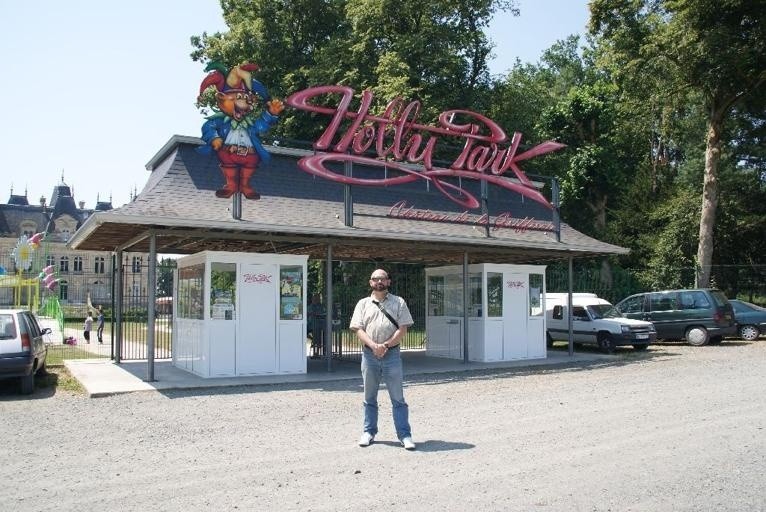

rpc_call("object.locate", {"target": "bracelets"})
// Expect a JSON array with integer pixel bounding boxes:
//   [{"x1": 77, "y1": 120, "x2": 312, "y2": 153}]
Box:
[{"x1": 383, "y1": 343, "x2": 389, "y2": 349}]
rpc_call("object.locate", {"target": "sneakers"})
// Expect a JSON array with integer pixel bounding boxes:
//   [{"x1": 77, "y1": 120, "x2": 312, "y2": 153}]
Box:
[
  {"x1": 359, "y1": 432, "x2": 375, "y2": 446},
  {"x1": 400, "y1": 436, "x2": 416, "y2": 450}
]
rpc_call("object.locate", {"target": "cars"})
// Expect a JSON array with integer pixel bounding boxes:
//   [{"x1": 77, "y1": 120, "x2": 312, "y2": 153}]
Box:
[
  {"x1": 728, "y1": 300, "x2": 766, "y2": 340},
  {"x1": 0, "y1": 310, "x2": 52, "y2": 395}
]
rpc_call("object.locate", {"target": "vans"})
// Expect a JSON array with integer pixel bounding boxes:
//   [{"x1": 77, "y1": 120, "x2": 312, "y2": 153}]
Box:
[{"x1": 546, "y1": 288, "x2": 739, "y2": 353}]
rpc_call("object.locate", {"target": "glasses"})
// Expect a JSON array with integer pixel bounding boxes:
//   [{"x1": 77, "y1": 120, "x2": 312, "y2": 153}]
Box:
[{"x1": 368, "y1": 276, "x2": 389, "y2": 283}]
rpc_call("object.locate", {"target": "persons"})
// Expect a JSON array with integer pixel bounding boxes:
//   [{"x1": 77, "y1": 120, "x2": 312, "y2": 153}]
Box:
[
  {"x1": 307, "y1": 292, "x2": 326, "y2": 347},
  {"x1": 348, "y1": 268, "x2": 416, "y2": 450},
  {"x1": 82, "y1": 311, "x2": 94, "y2": 344},
  {"x1": 96, "y1": 310, "x2": 104, "y2": 345}
]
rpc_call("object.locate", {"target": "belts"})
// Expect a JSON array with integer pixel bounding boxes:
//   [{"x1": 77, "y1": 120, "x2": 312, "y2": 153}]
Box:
[{"x1": 363, "y1": 343, "x2": 400, "y2": 350}]
